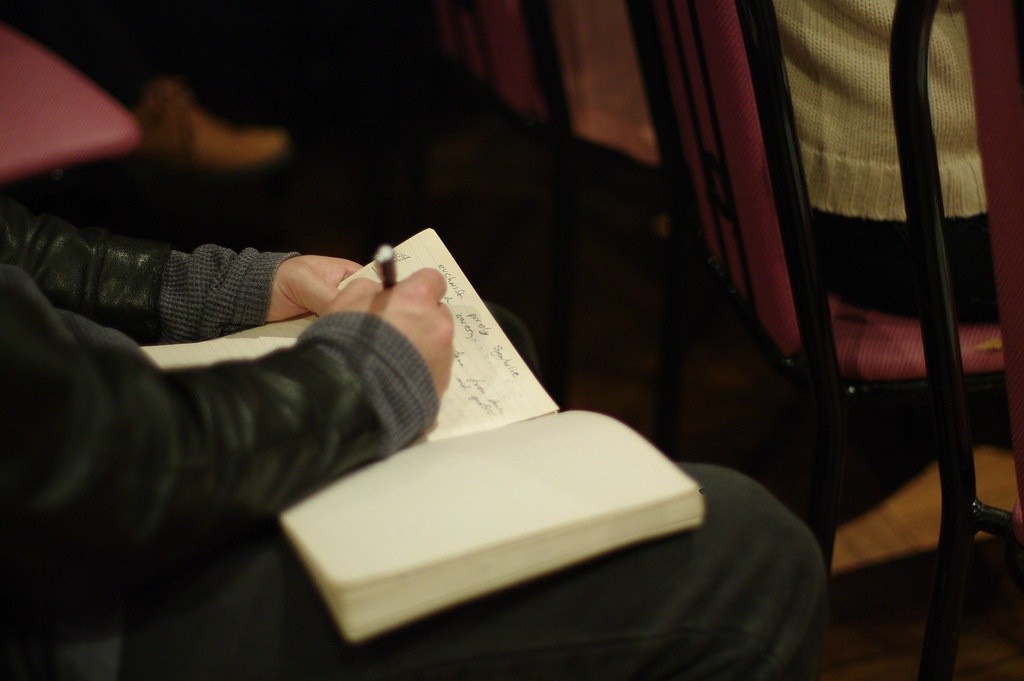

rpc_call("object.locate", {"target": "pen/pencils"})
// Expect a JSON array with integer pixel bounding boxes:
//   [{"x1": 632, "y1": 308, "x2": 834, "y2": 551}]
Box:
[{"x1": 373, "y1": 245, "x2": 398, "y2": 291}]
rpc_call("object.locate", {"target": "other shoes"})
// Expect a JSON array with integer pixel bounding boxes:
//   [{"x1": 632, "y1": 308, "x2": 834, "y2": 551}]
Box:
[{"x1": 120, "y1": 77, "x2": 289, "y2": 174}]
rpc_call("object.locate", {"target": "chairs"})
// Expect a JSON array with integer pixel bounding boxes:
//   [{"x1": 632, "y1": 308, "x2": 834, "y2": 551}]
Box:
[{"x1": 363, "y1": 0, "x2": 1024, "y2": 681}]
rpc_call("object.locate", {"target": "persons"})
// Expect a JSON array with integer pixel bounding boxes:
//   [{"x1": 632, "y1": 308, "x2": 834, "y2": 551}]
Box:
[
  {"x1": 774, "y1": 0, "x2": 1000, "y2": 324},
  {"x1": 0, "y1": 197, "x2": 828, "y2": 681}
]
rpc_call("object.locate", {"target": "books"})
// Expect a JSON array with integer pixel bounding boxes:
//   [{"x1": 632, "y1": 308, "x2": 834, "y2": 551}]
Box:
[{"x1": 141, "y1": 227, "x2": 704, "y2": 642}]
